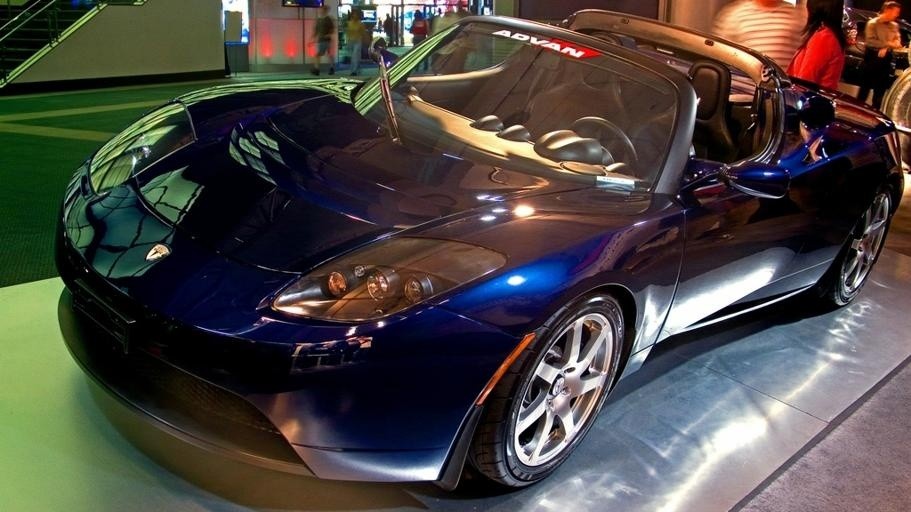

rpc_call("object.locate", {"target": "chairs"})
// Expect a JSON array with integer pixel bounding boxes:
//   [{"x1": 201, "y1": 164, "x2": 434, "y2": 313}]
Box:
[{"x1": 521, "y1": 32, "x2": 737, "y2": 179}]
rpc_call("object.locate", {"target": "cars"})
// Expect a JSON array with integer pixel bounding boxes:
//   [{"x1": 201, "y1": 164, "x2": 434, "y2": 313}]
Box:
[{"x1": 841, "y1": 4, "x2": 911, "y2": 77}]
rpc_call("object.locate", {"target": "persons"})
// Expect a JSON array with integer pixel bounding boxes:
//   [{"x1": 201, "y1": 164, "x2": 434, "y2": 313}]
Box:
[
  {"x1": 857, "y1": 1, "x2": 903, "y2": 112},
  {"x1": 711, "y1": 0, "x2": 809, "y2": 74},
  {"x1": 377, "y1": 0, "x2": 472, "y2": 75},
  {"x1": 308, "y1": 5, "x2": 336, "y2": 75},
  {"x1": 784, "y1": 0, "x2": 849, "y2": 91},
  {"x1": 344, "y1": 8, "x2": 365, "y2": 76}
]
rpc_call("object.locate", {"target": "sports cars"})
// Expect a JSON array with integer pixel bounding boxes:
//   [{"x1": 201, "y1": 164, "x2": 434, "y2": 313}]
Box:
[{"x1": 54, "y1": 9, "x2": 904, "y2": 491}]
